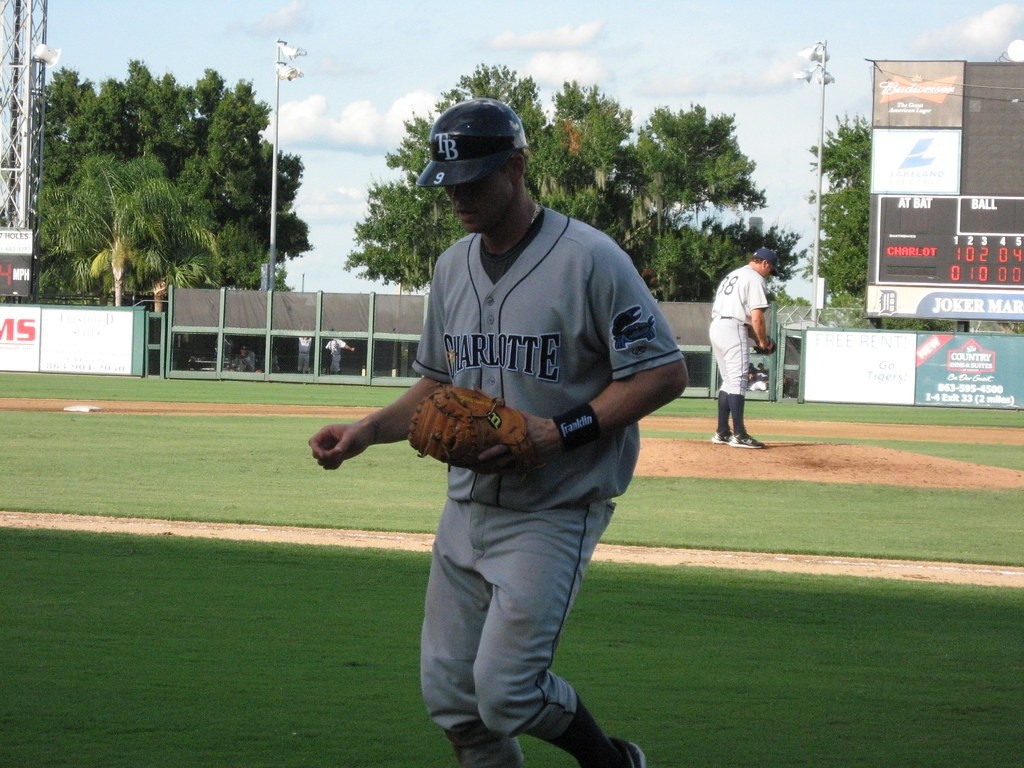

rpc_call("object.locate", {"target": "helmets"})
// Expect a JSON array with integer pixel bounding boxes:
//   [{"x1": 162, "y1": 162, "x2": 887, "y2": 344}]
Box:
[{"x1": 415, "y1": 98, "x2": 528, "y2": 187}]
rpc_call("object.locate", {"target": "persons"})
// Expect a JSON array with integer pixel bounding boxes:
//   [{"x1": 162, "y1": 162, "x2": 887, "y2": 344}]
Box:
[
  {"x1": 325, "y1": 338, "x2": 355, "y2": 374},
  {"x1": 297, "y1": 337, "x2": 312, "y2": 373},
  {"x1": 709, "y1": 247, "x2": 782, "y2": 449},
  {"x1": 239, "y1": 345, "x2": 248, "y2": 356},
  {"x1": 307, "y1": 99, "x2": 690, "y2": 768},
  {"x1": 746, "y1": 362, "x2": 769, "y2": 391}
]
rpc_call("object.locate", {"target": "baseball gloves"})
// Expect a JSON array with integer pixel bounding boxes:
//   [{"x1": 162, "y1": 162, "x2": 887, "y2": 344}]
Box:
[
  {"x1": 753, "y1": 336, "x2": 778, "y2": 356},
  {"x1": 406, "y1": 386, "x2": 537, "y2": 477}
]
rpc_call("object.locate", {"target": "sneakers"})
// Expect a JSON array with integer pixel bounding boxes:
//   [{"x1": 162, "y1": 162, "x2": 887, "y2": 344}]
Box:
[
  {"x1": 730, "y1": 434, "x2": 764, "y2": 448},
  {"x1": 712, "y1": 433, "x2": 733, "y2": 445}
]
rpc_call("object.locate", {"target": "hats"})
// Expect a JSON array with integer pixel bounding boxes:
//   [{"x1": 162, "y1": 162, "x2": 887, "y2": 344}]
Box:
[{"x1": 753, "y1": 249, "x2": 779, "y2": 276}]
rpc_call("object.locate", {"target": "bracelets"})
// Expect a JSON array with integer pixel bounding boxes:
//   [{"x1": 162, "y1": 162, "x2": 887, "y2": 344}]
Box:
[{"x1": 552, "y1": 403, "x2": 602, "y2": 453}]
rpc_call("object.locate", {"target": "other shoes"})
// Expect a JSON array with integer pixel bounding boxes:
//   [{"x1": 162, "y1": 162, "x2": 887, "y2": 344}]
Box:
[{"x1": 611, "y1": 737, "x2": 646, "y2": 768}]
